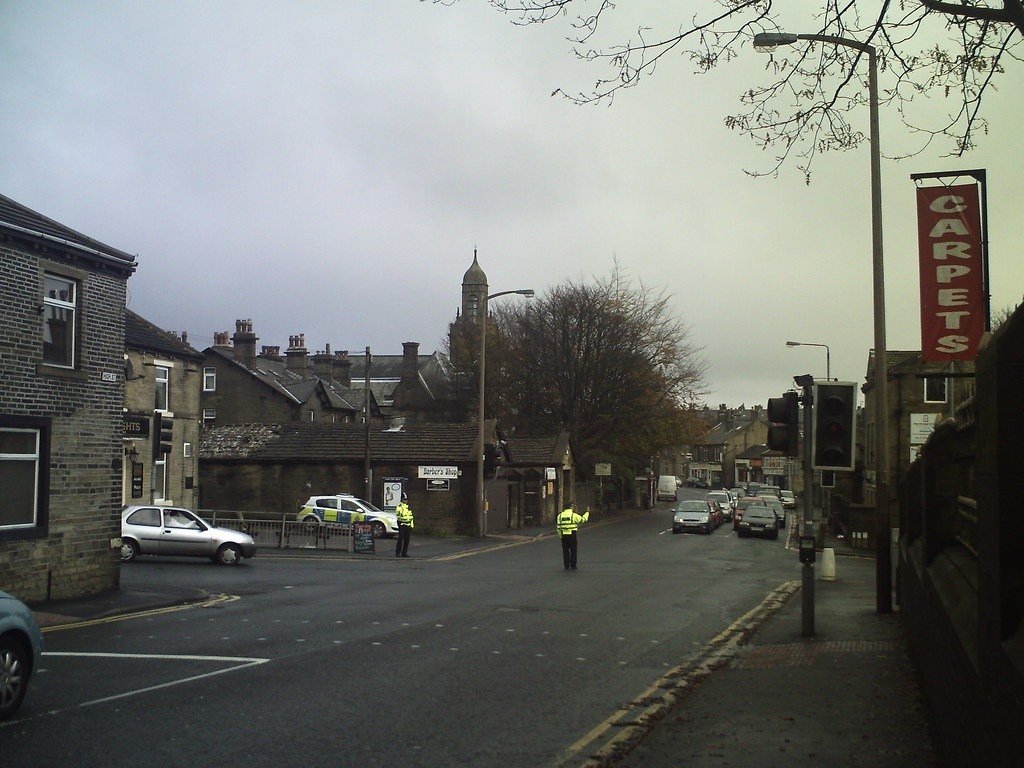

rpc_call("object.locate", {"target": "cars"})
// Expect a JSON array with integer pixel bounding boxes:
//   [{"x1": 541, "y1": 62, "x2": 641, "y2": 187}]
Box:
[
  {"x1": 297, "y1": 493, "x2": 400, "y2": 540},
  {"x1": 118, "y1": 505, "x2": 256, "y2": 567},
  {"x1": 0, "y1": 590, "x2": 45, "y2": 721},
  {"x1": 671, "y1": 481, "x2": 798, "y2": 539}
]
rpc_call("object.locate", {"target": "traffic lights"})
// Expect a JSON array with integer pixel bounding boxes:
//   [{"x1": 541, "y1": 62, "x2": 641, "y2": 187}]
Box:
[
  {"x1": 812, "y1": 382, "x2": 857, "y2": 470},
  {"x1": 766, "y1": 392, "x2": 799, "y2": 456}
]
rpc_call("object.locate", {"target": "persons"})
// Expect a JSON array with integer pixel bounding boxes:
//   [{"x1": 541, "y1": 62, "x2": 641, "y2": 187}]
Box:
[
  {"x1": 386, "y1": 487, "x2": 393, "y2": 505},
  {"x1": 557, "y1": 501, "x2": 590, "y2": 569},
  {"x1": 396, "y1": 493, "x2": 414, "y2": 557}
]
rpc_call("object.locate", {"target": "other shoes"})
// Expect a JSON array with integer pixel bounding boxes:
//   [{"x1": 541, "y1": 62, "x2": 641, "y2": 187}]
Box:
[
  {"x1": 565, "y1": 566, "x2": 577, "y2": 569},
  {"x1": 396, "y1": 555, "x2": 409, "y2": 558}
]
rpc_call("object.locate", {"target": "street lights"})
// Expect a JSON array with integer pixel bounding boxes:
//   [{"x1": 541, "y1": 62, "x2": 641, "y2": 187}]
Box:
[
  {"x1": 752, "y1": 32, "x2": 893, "y2": 615},
  {"x1": 473, "y1": 289, "x2": 536, "y2": 537},
  {"x1": 786, "y1": 341, "x2": 830, "y2": 381}
]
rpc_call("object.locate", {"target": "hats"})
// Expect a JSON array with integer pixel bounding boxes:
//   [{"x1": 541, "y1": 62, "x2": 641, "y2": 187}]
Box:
[
  {"x1": 564, "y1": 501, "x2": 573, "y2": 509},
  {"x1": 401, "y1": 493, "x2": 408, "y2": 500}
]
